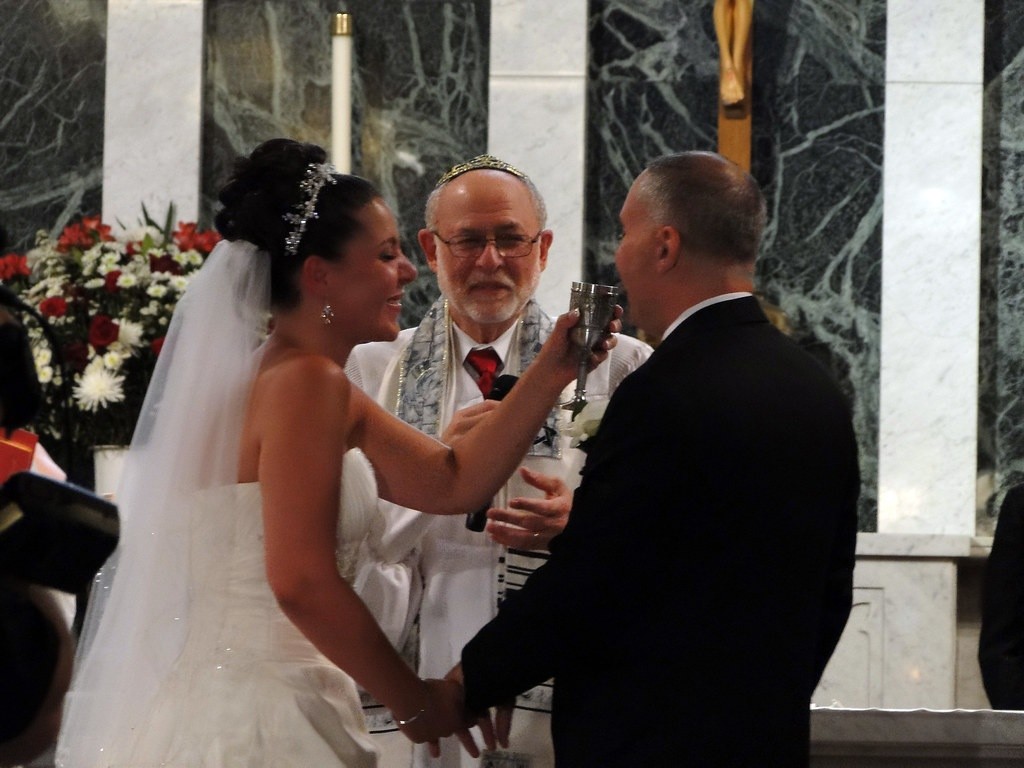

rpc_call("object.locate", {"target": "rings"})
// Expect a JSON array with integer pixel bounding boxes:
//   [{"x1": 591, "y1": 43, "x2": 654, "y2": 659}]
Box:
[{"x1": 531, "y1": 531, "x2": 539, "y2": 545}]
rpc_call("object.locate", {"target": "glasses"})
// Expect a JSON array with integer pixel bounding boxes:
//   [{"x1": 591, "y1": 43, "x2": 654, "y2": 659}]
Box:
[{"x1": 432, "y1": 229, "x2": 542, "y2": 259}]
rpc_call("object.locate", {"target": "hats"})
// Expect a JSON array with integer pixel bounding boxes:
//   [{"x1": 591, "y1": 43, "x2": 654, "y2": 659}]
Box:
[{"x1": 435, "y1": 153, "x2": 528, "y2": 188}]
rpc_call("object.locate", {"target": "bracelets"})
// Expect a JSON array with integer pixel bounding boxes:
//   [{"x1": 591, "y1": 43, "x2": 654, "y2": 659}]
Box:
[{"x1": 392, "y1": 680, "x2": 427, "y2": 725}]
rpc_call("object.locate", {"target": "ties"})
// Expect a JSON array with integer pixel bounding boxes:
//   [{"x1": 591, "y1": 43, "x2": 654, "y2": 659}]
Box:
[{"x1": 465, "y1": 350, "x2": 501, "y2": 400}]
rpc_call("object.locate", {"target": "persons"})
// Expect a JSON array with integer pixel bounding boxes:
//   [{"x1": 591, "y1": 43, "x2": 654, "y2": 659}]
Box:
[
  {"x1": 55, "y1": 138, "x2": 623, "y2": 768},
  {"x1": 344, "y1": 156, "x2": 657, "y2": 768},
  {"x1": 430, "y1": 151, "x2": 861, "y2": 768}
]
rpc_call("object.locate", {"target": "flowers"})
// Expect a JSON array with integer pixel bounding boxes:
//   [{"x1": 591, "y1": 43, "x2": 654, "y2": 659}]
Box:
[
  {"x1": 555, "y1": 395, "x2": 610, "y2": 453},
  {"x1": 0, "y1": 201, "x2": 222, "y2": 439}
]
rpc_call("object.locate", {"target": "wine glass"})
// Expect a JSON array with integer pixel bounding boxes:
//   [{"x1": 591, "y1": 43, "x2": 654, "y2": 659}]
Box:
[{"x1": 554, "y1": 282, "x2": 617, "y2": 412}]
